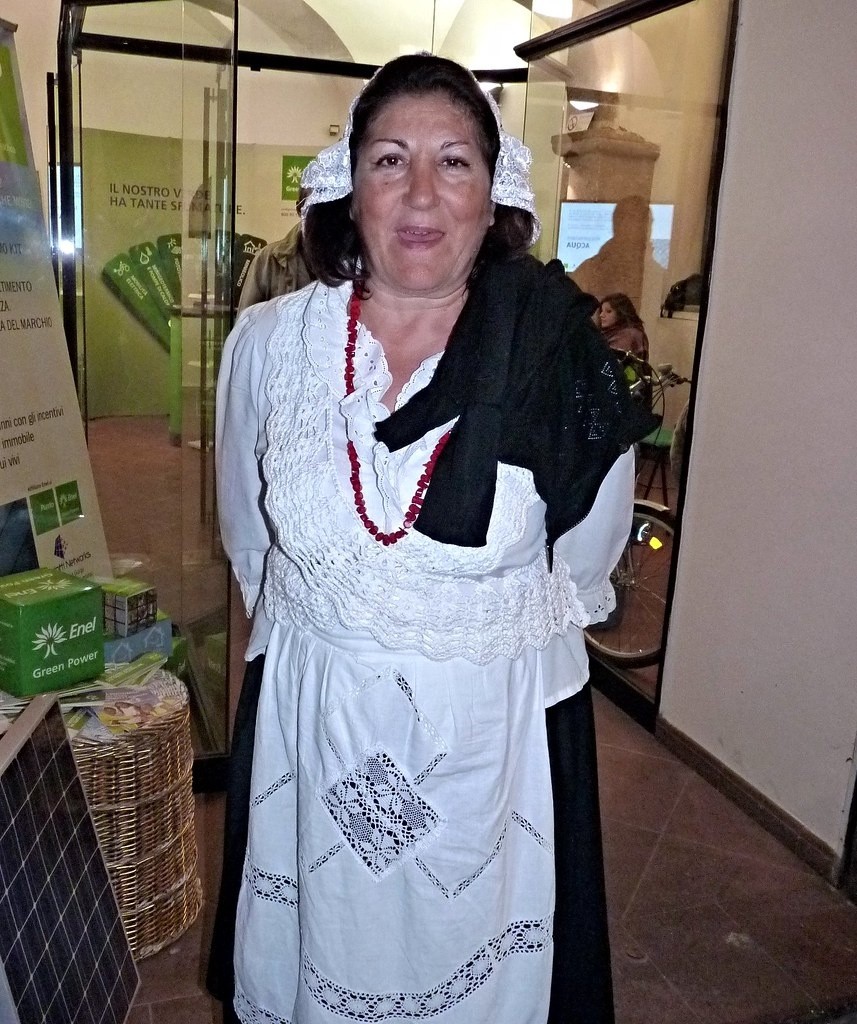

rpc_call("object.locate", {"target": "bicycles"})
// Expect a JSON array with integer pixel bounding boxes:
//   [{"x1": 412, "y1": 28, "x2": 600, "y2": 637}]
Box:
[{"x1": 583, "y1": 347, "x2": 691, "y2": 670}]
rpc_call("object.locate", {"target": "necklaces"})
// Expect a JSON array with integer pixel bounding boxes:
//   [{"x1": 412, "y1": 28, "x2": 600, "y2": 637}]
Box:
[{"x1": 342, "y1": 273, "x2": 456, "y2": 547}]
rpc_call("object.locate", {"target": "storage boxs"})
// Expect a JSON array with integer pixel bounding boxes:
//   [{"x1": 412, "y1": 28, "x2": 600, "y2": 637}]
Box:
[
  {"x1": 0, "y1": 567, "x2": 105, "y2": 697},
  {"x1": 202, "y1": 633, "x2": 228, "y2": 693},
  {"x1": 104, "y1": 611, "x2": 175, "y2": 660},
  {"x1": 158, "y1": 637, "x2": 186, "y2": 679}
]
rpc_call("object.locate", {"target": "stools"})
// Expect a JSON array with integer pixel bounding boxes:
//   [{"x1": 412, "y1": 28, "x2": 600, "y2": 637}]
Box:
[{"x1": 631, "y1": 429, "x2": 675, "y2": 538}]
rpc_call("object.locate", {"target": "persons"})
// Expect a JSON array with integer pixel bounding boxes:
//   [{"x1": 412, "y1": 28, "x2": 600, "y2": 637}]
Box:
[{"x1": 206, "y1": 50, "x2": 651, "y2": 1023}]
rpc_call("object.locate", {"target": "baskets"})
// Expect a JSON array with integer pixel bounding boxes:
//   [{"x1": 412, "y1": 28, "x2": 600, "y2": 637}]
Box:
[{"x1": 0, "y1": 663, "x2": 206, "y2": 962}]
